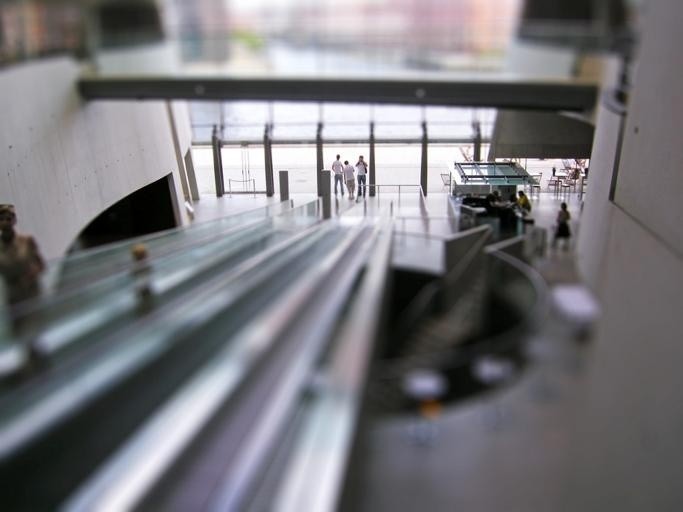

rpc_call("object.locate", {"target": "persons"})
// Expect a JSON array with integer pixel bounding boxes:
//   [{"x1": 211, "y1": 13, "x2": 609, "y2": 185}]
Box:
[
  {"x1": 0, "y1": 204, "x2": 46, "y2": 359},
  {"x1": 331, "y1": 154, "x2": 344, "y2": 196},
  {"x1": 343, "y1": 160, "x2": 355, "y2": 200},
  {"x1": 355, "y1": 155, "x2": 367, "y2": 197},
  {"x1": 129, "y1": 243, "x2": 153, "y2": 312},
  {"x1": 552, "y1": 202, "x2": 572, "y2": 246},
  {"x1": 463, "y1": 190, "x2": 531, "y2": 219}
]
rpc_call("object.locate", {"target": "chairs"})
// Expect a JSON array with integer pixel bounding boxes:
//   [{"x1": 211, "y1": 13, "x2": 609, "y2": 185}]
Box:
[{"x1": 530, "y1": 163, "x2": 589, "y2": 202}]
